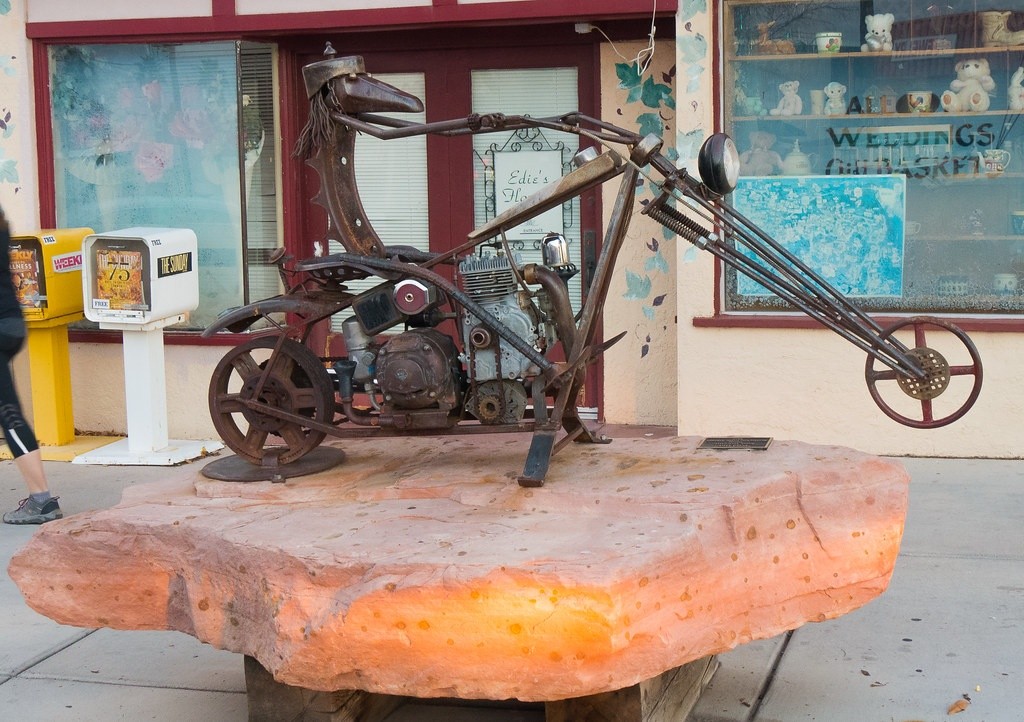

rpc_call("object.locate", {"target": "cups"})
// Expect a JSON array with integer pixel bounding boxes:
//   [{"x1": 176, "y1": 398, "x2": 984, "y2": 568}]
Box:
[
  {"x1": 1011, "y1": 211, "x2": 1024, "y2": 235},
  {"x1": 816, "y1": 32, "x2": 842, "y2": 54},
  {"x1": 810, "y1": 89, "x2": 825, "y2": 115},
  {"x1": 905, "y1": 221, "x2": 921, "y2": 236},
  {"x1": 907, "y1": 91, "x2": 932, "y2": 113},
  {"x1": 743, "y1": 97, "x2": 761, "y2": 116}
]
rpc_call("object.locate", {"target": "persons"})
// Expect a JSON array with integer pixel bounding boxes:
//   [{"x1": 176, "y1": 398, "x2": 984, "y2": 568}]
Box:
[{"x1": 1, "y1": 197, "x2": 63, "y2": 527}]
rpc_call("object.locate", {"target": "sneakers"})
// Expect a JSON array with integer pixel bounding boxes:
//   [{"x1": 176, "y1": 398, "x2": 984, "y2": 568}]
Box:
[{"x1": 3, "y1": 496, "x2": 63, "y2": 524}]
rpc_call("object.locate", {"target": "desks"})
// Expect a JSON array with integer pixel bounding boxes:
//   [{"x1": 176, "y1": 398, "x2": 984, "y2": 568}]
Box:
[{"x1": 1, "y1": 426, "x2": 913, "y2": 722}]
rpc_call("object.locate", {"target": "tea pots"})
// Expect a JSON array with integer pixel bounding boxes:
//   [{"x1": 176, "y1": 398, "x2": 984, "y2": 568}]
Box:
[
  {"x1": 977, "y1": 149, "x2": 1011, "y2": 174},
  {"x1": 777, "y1": 139, "x2": 819, "y2": 176}
]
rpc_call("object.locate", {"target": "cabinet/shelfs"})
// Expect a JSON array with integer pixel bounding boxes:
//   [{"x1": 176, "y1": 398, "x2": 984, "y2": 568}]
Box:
[{"x1": 723, "y1": 0, "x2": 1024, "y2": 315}]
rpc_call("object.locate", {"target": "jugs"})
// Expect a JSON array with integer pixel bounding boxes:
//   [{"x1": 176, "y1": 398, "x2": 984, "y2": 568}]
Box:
[{"x1": 541, "y1": 234, "x2": 572, "y2": 267}]
